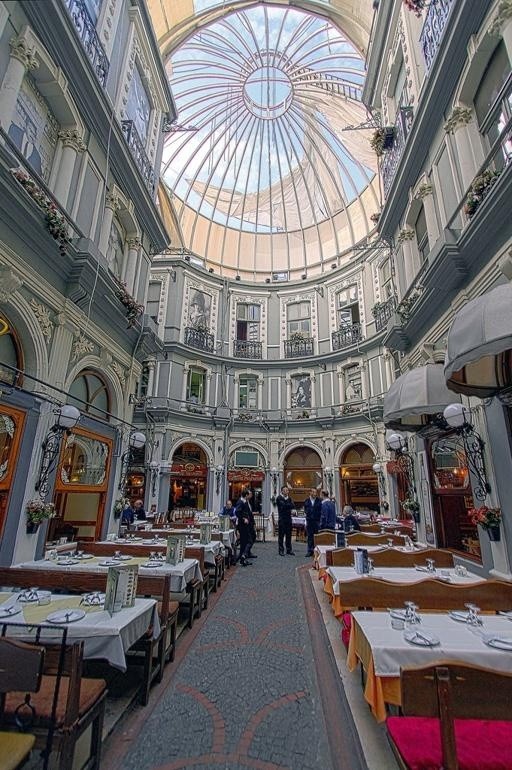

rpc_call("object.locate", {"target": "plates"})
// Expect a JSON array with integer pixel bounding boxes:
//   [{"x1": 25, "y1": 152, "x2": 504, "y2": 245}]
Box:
[
  {"x1": 57, "y1": 547, "x2": 169, "y2": 568},
  {"x1": 1, "y1": 585, "x2": 112, "y2": 627},
  {"x1": 387, "y1": 601, "x2": 511, "y2": 656}
]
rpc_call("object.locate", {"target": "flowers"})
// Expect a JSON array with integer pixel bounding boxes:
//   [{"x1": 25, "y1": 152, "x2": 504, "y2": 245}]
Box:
[
  {"x1": 112, "y1": 497, "x2": 130, "y2": 513},
  {"x1": 462, "y1": 167, "x2": 496, "y2": 221},
  {"x1": 400, "y1": 498, "x2": 420, "y2": 513},
  {"x1": 11, "y1": 171, "x2": 72, "y2": 258},
  {"x1": 379, "y1": 500, "x2": 389, "y2": 508},
  {"x1": 24, "y1": 498, "x2": 57, "y2": 525},
  {"x1": 467, "y1": 505, "x2": 503, "y2": 529},
  {"x1": 109, "y1": 274, "x2": 143, "y2": 333}
]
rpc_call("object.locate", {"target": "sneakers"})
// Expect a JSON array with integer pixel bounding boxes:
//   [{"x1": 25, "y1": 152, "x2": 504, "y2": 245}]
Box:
[
  {"x1": 279, "y1": 550, "x2": 296, "y2": 556},
  {"x1": 239, "y1": 552, "x2": 257, "y2": 566}
]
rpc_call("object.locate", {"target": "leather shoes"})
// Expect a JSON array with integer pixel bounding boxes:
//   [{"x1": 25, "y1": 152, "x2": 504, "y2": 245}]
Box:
[{"x1": 304, "y1": 550, "x2": 314, "y2": 558}]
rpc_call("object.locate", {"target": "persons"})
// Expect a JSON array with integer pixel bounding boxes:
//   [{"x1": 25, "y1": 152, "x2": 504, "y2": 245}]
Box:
[
  {"x1": 121, "y1": 499, "x2": 147, "y2": 525},
  {"x1": 276, "y1": 486, "x2": 297, "y2": 556},
  {"x1": 318, "y1": 489, "x2": 336, "y2": 531},
  {"x1": 304, "y1": 487, "x2": 322, "y2": 557},
  {"x1": 219, "y1": 488, "x2": 258, "y2": 566},
  {"x1": 336, "y1": 505, "x2": 362, "y2": 547}
]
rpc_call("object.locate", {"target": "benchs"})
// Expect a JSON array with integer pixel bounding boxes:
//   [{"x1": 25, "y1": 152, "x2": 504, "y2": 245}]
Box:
[
  {"x1": 318, "y1": 545, "x2": 510, "y2": 653},
  {"x1": 313, "y1": 513, "x2": 418, "y2": 547},
  {"x1": 383, "y1": 659, "x2": 510, "y2": 768}
]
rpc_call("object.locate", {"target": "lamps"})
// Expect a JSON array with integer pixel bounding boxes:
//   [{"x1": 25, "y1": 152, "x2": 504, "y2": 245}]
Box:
[
  {"x1": 149, "y1": 461, "x2": 159, "y2": 497},
  {"x1": 374, "y1": 464, "x2": 387, "y2": 496},
  {"x1": 119, "y1": 430, "x2": 147, "y2": 497},
  {"x1": 324, "y1": 466, "x2": 334, "y2": 499},
  {"x1": 431, "y1": 401, "x2": 494, "y2": 502},
  {"x1": 32, "y1": 403, "x2": 80, "y2": 497},
  {"x1": 268, "y1": 467, "x2": 279, "y2": 496},
  {"x1": 214, "y1": 464, "x2": 225, "y2": 495},
  {"x1": 385, "y1": 432, "x2": 418, "y2": 494}
]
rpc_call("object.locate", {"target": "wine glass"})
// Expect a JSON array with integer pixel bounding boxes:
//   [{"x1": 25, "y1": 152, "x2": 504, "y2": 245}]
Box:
[
  {"x1": 119, "y1": 509, "x2": 199, "y2": 545},
  {"x1": 378, "y1": 520, "x2": 468, "y2": 577}
]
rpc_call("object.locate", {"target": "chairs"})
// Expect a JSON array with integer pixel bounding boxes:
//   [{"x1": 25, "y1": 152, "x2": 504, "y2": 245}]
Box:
[
  {"x1": 0, "y1": 623, "x2": 108, "y2": 768},
  {"x1": 252, "y1": 510, "x2": 279, "y2": 543},
  {"x1": 1, "y1": 552, "x2": 209, "y2": 708},
  {"x1": 80, "y1": 512, "x2": 239, "y2": 594}
]
